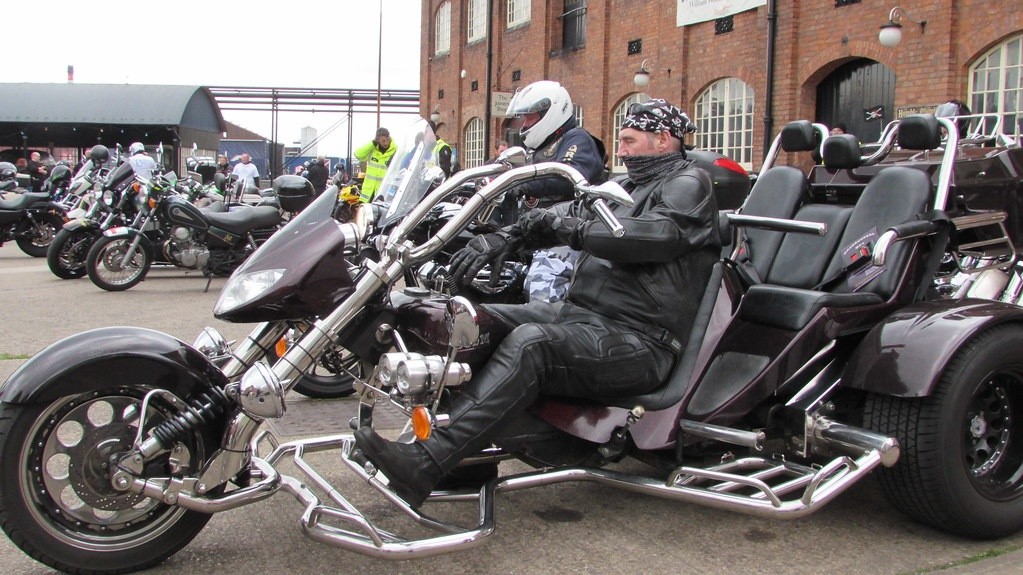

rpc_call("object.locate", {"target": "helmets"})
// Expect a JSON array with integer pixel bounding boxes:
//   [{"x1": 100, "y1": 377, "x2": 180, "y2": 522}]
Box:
[
  {"x1": 90, "y1": 145, "x2": 111, "y2": 163},
  {"x1": 942, "y1": 98, "x2": 969, "y2": 137},
  {"x1": 131, "y1": 143, "x2": 146, "y2": 154},
  {"x1": 512, "y1": 80, "x2": 574, "y2": 150},
  {"x1": 52, "y1": 166, "x2": 71, "y2": 183}
]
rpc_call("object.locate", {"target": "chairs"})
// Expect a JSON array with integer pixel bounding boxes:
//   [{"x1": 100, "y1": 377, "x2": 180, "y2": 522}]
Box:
[{"x1": 526, "y1": 215, "x2": 739, "y2": 450}]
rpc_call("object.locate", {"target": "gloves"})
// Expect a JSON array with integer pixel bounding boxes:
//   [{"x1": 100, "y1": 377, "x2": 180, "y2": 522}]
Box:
[
  {"x1": 511, "y1": 210, "x2": 584, "y2": 250},
  {"x1": 446, "y1": 220, "x2": 523, "y2": 287}
]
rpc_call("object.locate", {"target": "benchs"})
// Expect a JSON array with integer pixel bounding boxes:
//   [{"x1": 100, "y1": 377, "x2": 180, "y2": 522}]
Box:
[{"x1": 719, "y1": 116, "x2": 959, "y2": 331}]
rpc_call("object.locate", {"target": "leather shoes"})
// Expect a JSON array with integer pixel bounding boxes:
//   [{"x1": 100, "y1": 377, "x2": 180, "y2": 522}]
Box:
[
  {"x1": 353, "y1": 426, "x2": 440, "y2": 510},
  {"x1": 428, "y1": 464, "x2": 497, "y2": 493}
]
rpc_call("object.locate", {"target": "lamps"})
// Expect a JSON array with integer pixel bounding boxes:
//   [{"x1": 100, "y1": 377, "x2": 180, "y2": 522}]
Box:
[
  {"x1": 431, "y1": 104, "x2": 454, "y2": 122},
  {"x1": 634, "y1": 59, "x2": 670, "y2": 87},
  {"x1": 878, "y1": 6, "x2": 926, "y2": 46}
]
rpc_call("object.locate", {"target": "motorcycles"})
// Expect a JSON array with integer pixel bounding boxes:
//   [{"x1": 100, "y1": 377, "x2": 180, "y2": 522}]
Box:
[
  {"x1": 324, "y1": 171, "x2": 362, "y2": 223},
  {"x1": 0, "y1": 142, "x2": 316, "y2": 292},
  {"x1": 213, "y1": 119, "x2": 750, "y2": 398},
  {"x1": 0, "y1": 115, "x2": 1023, "y2": 575}
]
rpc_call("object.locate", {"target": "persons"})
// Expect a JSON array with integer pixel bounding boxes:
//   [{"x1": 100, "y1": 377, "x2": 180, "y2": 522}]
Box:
[
  {"x1": 15, "y1": 150, "x2": 91, "y2": 192},
  {"x1": 216, "y1": 153, "x2": 260, "y2": 189},
  {"x1": 295, "y1": 156, "x2": 345, "y2": 199},
  {"x1": 490, "y1": 80, "x2": 609, "y2": 222},
  {"x1": 811, "y1": 124, "x2": 863, "y2": 165},
  {"x1": 354, "y1": 97, "x2": 721, "y2": 510},
  {"x1": 354, "y1": 119, "x2": 452, "y2": 205},
  {"x1": 485, "y1": 140, "x2": 509, "y2": 165},
  {"x1": 940, "y1": 99, "x2": 971, "y2": 140},
  {"x1": 128, "y1": 142, "x2": 157, "y2": 187}
]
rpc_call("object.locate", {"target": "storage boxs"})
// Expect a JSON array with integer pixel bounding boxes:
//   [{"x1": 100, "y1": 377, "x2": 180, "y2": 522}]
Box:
[
  {"x1": 272, "y1": 175, "x2": 316, "y2": 213},
  {"x1": 185, "y1": 156, "x2": 217, "y2": 182},
  {"x1": 686, "y1": 151, "x2": 750, "y2": 209}
]
